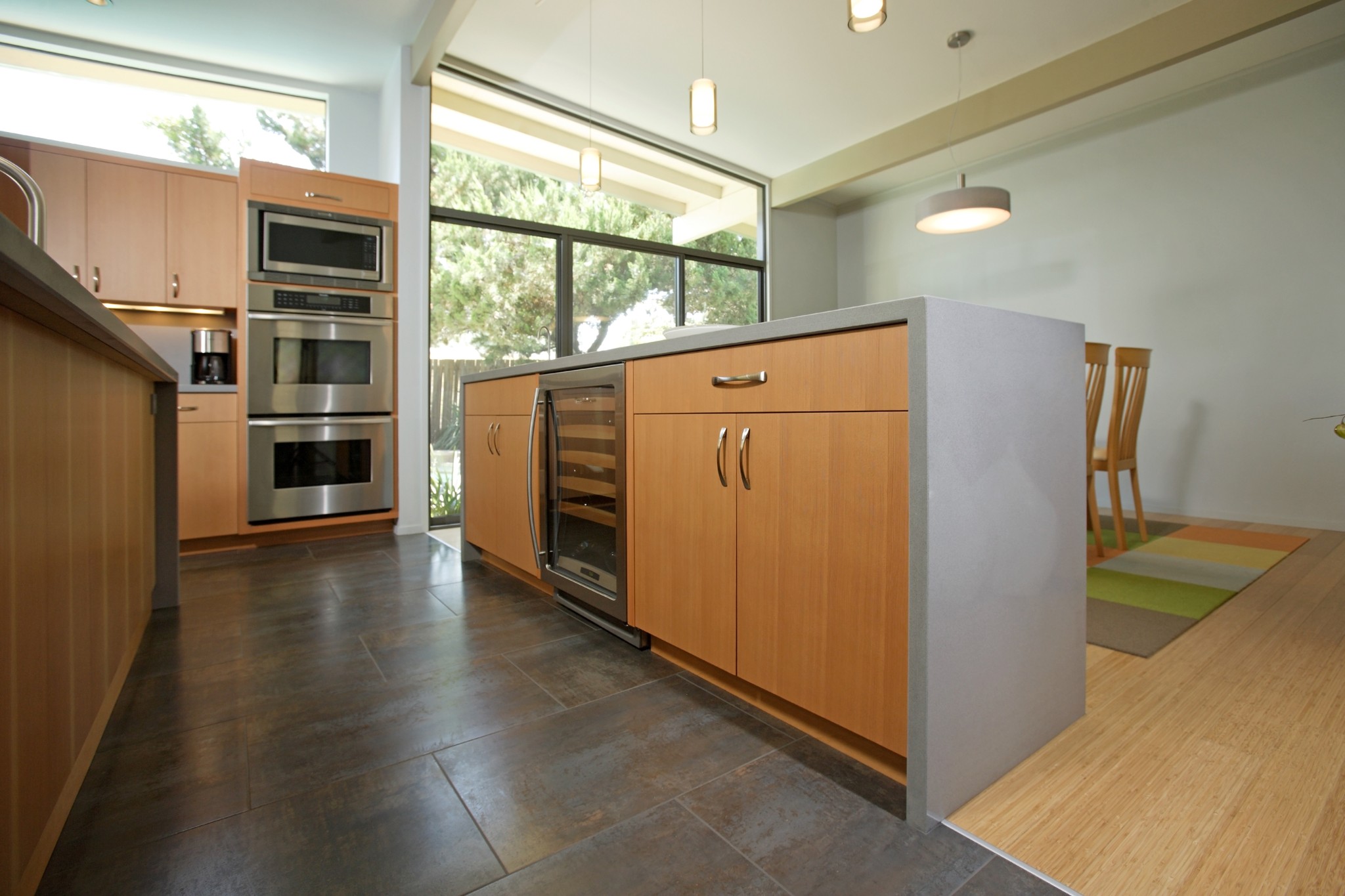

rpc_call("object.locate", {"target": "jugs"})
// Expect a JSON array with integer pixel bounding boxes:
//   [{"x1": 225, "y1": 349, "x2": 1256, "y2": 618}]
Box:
[{"x1": 198, "y1": 352, "x2": 224, "y2": 380}]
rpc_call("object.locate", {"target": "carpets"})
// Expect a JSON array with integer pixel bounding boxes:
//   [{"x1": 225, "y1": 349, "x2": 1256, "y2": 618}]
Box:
[{"x1": 1084, "y1": 513, "x2": 1311, "y2": 659}]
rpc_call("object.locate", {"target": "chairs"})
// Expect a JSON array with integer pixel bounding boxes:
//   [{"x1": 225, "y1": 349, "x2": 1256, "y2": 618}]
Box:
[{"x1": 1083, "y1": 342, "x2": 1153, "y2": 557}]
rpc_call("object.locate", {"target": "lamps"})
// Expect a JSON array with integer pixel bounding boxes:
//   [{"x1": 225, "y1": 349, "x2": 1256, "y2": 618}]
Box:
[
  {"x1": 580, "y1": 0, "x2": 601, "y2": 192},
  {"x1": 691, "y1": 0, "x2": 717, "y2": 136},
  {"x1": 848, "y1": 0, "x2": 886, "y2": 32},
  {"x1": 915, "y1": 174, "x2": 1011, "y2": 233}
]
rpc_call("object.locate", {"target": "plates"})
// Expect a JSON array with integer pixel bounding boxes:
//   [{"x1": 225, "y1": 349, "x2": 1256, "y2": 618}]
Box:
[{"x1": 663, "y1": 323, "x2": 741, "y2": 340}]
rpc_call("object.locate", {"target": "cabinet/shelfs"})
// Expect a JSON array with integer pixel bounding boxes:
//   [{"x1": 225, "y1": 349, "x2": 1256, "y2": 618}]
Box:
[
  {"x1": 0, "y1": 134, "x2": 238, "y2": 314},
  {"x1": 176, "y1": 391, "x2": 237, "y2": 541},
  {"x1": 242, "y1": 158, "x2": 395, "y2": 218},
  {"x1": 461, "y1": 317, "x2": 1087, "y2": 833}
]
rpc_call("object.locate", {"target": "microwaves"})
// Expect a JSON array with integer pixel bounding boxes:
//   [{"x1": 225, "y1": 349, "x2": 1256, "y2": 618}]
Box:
[{"x1": 245, "y1": 199, "x2": 395, "y2": 292}]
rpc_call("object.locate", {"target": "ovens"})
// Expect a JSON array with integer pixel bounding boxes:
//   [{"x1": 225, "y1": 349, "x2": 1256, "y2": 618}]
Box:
[{"x1": 245, "y1": 284, "x2": 394, "y2": 521}]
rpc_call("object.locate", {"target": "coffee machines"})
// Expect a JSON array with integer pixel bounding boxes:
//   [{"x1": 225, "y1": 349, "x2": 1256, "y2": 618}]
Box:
[{"x1": 191, "y1": 329, "x2": 232, "y2": 384}]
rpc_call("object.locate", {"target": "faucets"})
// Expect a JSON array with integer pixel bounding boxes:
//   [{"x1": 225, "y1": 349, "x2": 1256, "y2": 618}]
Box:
[{"x1": 0, "y1": 156, "x2": 46, "y2": 248}]
